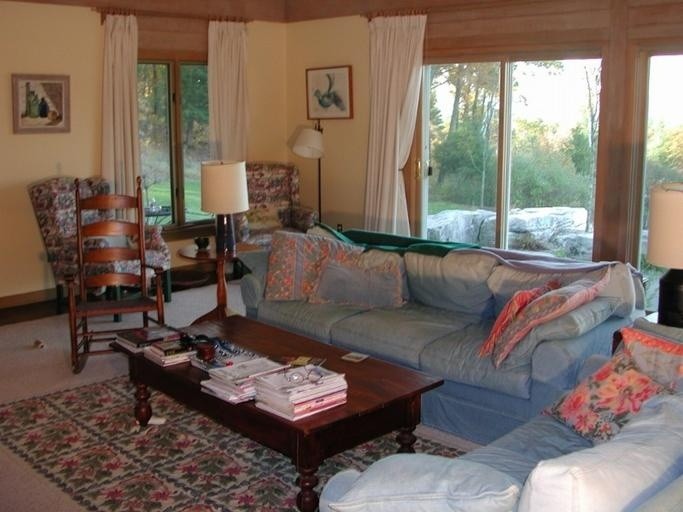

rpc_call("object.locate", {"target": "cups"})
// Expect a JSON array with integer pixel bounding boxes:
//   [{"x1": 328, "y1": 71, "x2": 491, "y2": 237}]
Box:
[
  {"x1": 196, "y1": 344, "x2": 217, "y2": 361},
  {"x1": 193, "y1": 237, "x2": 209, "y2": 249}
]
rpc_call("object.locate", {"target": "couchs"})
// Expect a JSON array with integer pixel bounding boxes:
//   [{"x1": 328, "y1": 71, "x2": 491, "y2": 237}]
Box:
[
  {"x1": 236, "y1": 228, "x2": 652, "y2": 445},
  {"x1": 317, "y1": 316, "x2": 683, "y2": 512}
]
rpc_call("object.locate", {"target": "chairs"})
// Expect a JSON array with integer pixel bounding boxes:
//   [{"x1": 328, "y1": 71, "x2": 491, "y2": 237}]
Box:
[
  {"x1": 61, "y1": 176, "x2": 164, "y2": 373},
  {"x1": 25, "y1": 174, "x2": 173, "y2": 323},
  {"x1": 226, "y1": 160, "x2": 318, "y2": 261}
]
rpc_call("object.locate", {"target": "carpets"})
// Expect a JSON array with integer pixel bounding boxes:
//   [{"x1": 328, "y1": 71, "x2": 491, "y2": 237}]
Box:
[{"x1": 0, "y1": 372, "x2": 466, "y2": 511}]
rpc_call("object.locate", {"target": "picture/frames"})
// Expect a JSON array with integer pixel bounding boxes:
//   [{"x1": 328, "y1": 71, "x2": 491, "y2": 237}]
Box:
[
  {"x1": 304, "y1": 66, "x2": 353, "y2": 121},
  {"x1": 9, "y1": 73, "x2": 71, "y2": 134}
]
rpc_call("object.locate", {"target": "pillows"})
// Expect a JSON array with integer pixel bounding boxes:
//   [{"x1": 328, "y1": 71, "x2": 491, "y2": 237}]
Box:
[
  {"x1": 403, "y1": 243, "x2": 499, "y2": 314},
  {"x1": 331, "y1": 449, "x2": 522, "y2": 512},
  {"x1": 620, "y1": 325, "x2": 683, "y2": 393},
  {"x1": 307, "y1": 252, "x2": 407, "y2": 313},
  {"x1": 264, "y1": 234, "x2": 362, "y2": 302},
  {"x1": 479, "y1": 259, "x2": 631, "y2": 371},
  {"x1": 541, "y1": 349, "x2": 664, "y2": 445},
  {"x1": 363, "y1": 249, "x2": 410, "y2": 301}
]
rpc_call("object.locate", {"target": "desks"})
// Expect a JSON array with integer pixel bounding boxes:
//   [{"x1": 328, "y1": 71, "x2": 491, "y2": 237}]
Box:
[
  {"x1": 109, "y1": 314, "x2": 445, "y2": 511},
  {"x1": 178, "y1": 243, "x2": 238, "y2": 325}
]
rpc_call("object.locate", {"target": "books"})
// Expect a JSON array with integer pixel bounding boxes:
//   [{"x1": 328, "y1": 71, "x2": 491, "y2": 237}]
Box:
[{"x1": 114, "y1": 323, "x2": 369, "y2": 421}]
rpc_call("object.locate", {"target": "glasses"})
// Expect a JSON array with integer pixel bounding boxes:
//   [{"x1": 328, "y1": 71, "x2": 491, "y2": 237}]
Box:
[{"x1": 285, "y1": 365, "x2": 325, "y2": 385}]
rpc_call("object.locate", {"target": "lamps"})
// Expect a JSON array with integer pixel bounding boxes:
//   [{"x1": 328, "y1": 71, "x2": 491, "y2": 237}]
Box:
[
  {"x1": 191, "y1": 159, "x2": 249, "y2": 324},
  {"x1": 287, "y1": 121, "x2": 324, "y2": 222},
  {"x1": 646, "y1": 185, "x2": 683, "y2": 324}
]
